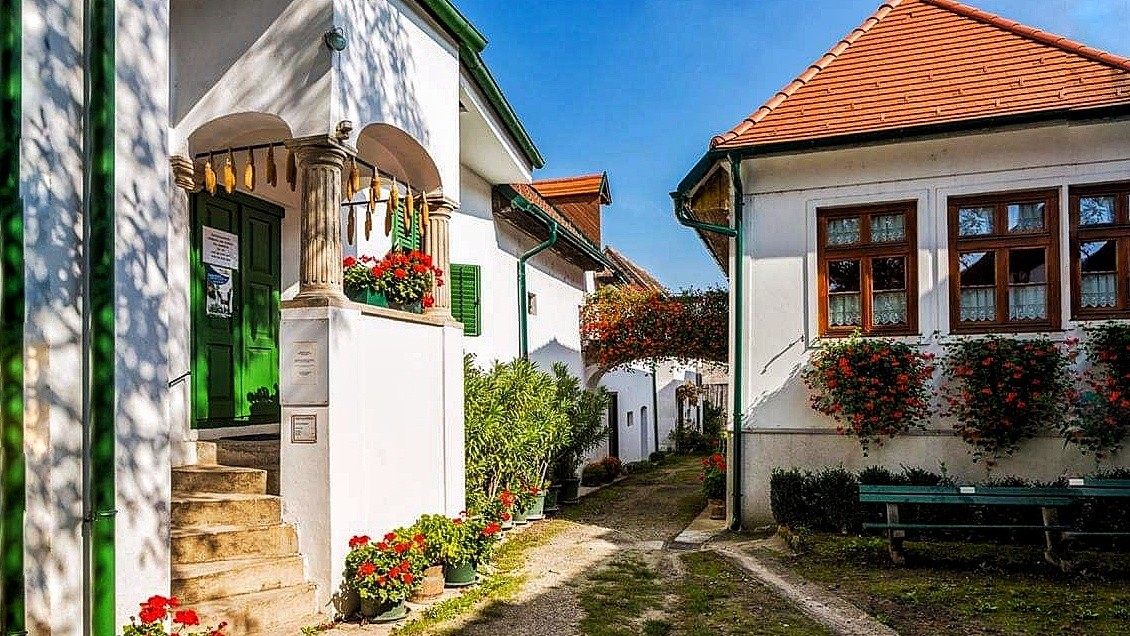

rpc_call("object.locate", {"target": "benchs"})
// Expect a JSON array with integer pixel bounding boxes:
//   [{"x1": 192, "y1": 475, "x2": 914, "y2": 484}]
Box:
[{"x1": 859, "y1": 480, "x2": 1130, "y2": 572}]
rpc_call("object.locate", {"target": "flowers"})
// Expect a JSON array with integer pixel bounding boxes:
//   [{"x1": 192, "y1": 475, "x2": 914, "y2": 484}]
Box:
[
  {"x1": 345, "y1": 481, "x2": 541, "y2": 605},
  {"x1": 580, "y1": 281, "x2": 730, "y2": 376},
  {"x1": 344, "y1": 247, "x2": 445, "y2": 309},
  {"x1": 123, "y1": 596, "x2": 227, "y2": 636},
  {"x1": 697, "y1": 452, "x2": 727, "y2": 501},
  {"x1": 801, "y1": 314, "x2": 1130, "y2": 481},
  {"x1": 585, "y1": 455, "x2": 622, "y2": 474}
]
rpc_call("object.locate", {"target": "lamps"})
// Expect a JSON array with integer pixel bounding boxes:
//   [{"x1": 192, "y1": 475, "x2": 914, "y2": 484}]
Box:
[{"x1": 325, "y1": 25, "x2": 345, "y2": 51}]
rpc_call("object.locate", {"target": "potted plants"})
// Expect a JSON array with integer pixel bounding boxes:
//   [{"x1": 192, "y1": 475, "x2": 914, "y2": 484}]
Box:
[{"x1": 463, "y1": 351, "x2": 615, "y2": 526}]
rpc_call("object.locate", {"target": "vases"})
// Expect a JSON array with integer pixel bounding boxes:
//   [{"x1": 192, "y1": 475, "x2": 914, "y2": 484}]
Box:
[
  {"x1": 707, "y1": 499, "x2": 726, "y2": 520},
  {"x1": 583, "y1": 469, "x2": 621, "y2": 487},
  {"x1": 345, "y1": 282, "x2": 422, "y2": 313},
  {"x1": 359, "y1": 554, "x2": 476, "y2": 618}
]
